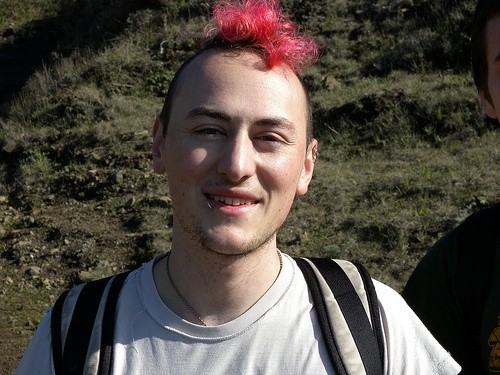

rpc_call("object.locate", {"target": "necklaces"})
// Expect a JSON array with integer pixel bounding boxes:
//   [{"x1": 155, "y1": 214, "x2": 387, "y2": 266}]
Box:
[{"x1": 167, "y1": 249, "x2": 283, "y2": 327}]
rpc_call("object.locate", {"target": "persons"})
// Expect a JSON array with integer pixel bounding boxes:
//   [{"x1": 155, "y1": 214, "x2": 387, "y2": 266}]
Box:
[
  {"x1": 402, "y1": 0, "x2": 500, "y2": 375},
  {"x1": 12, "y1": 0, "x2": 464, "y2": 375}
]
